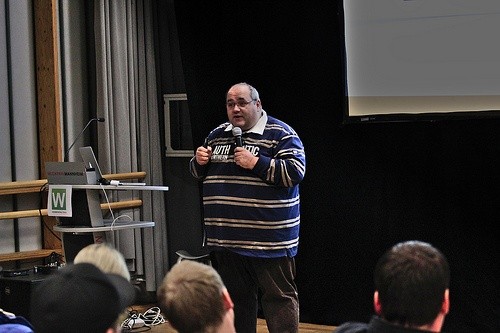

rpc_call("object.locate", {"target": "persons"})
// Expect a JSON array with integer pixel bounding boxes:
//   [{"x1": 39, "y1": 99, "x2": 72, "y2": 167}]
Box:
[
  {"x1": 0, "y1": 241, "x2": 136, "y2": 333},
  {"x1": 188, "y1": 82, "x2": 306, "y2": 332},
  {"x1": 158, "y1": 260, "x2": 237, "y2": 333},
  {"x1": 327, "y1": 241, "x2": 451, "y2": 333}
]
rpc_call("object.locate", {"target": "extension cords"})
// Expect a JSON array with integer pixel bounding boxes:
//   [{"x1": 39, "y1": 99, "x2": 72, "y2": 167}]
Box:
[{"x1": 33, "y1": 264, "x2": 58, "y2": 274}]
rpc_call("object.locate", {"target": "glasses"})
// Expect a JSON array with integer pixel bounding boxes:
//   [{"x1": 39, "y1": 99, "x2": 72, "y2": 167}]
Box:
[{"x1": 227, "y1": 99, "x2": 254, "y2": 107}]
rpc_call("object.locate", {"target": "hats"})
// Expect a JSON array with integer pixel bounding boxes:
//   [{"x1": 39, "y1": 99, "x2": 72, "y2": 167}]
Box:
[{"x1": 31, "y1": 262, "x2": 135, "y2": 333}]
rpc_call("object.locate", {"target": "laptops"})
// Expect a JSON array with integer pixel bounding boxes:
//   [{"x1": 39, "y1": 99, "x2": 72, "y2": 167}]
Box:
[{"x1": 79, "y1": 146, "x2": 146, "y2": 186}]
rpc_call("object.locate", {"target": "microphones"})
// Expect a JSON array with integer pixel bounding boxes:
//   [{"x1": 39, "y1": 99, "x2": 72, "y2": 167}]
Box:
[
  {"x1": 64, "y1": 118, "x2": 105, "y2": 162},
  {"x1": 232, "y1": 126, "x2": 243, "y2": 147}
]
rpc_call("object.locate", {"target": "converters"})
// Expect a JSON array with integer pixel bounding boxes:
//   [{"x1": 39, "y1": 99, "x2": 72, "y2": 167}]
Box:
[{"x1": 125, "y1": 317, "x2": 145, "y2": 328}]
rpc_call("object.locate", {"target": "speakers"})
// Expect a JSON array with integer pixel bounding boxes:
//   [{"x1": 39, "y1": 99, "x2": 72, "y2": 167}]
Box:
[{"x1": 0, "y1": 268, "x2": 49, "y2": 324}]
